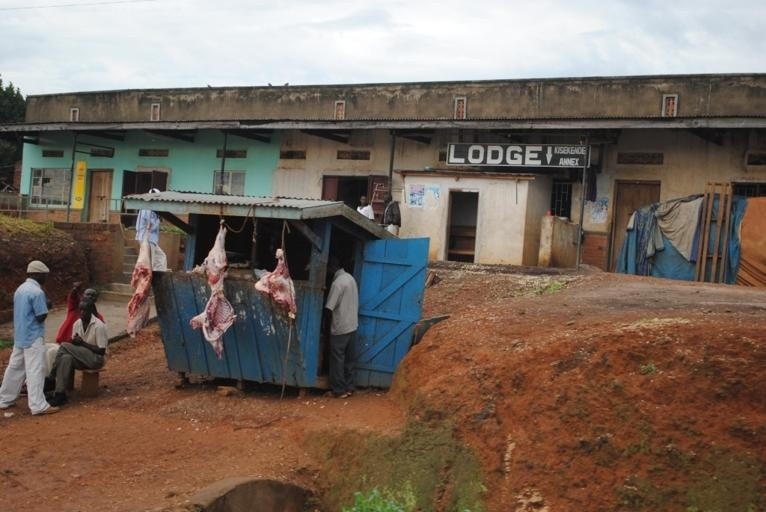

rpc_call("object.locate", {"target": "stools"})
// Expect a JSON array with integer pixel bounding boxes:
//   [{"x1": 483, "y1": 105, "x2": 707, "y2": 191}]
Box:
[{"x1": 78, "y1": 367, "x2": 104, "y2": 398}]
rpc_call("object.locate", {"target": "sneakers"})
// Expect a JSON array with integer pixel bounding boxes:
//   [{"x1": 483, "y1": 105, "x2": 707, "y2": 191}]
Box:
[
  {"x1": 40, "y1": 406, "x2": 59, "y2": 413},
  {"x1": 328, "y1": 390, "x2": 348, "y2": 398}
]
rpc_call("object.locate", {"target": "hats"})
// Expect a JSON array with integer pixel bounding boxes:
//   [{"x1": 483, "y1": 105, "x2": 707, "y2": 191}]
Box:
[{"x1": 26, "y1": 260, "x2": 50, "y2": 273}]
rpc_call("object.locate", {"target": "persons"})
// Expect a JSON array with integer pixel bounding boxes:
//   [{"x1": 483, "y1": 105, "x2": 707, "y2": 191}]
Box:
[
  {"x1": 0, "y1": 257, "x2": 60, "y2": 415},
  {"x1": 19, "y1": 281, "x2": 105, "y2": 395},
  {"x1": 322, "y1": 251, "x2": 360, "y2": 401},
  {"x1": 378, "y1": 192, "x2": 401, "y2": 238},
  {"x1": 355, "y1": 195, "x2": 374, "y2": 223},
  {"x1": 135, "y1": 188, "x2": 167, "y2": 295},
  {"x1": 43, "y1": 298, "x2": 107, "y2": 407}
]
